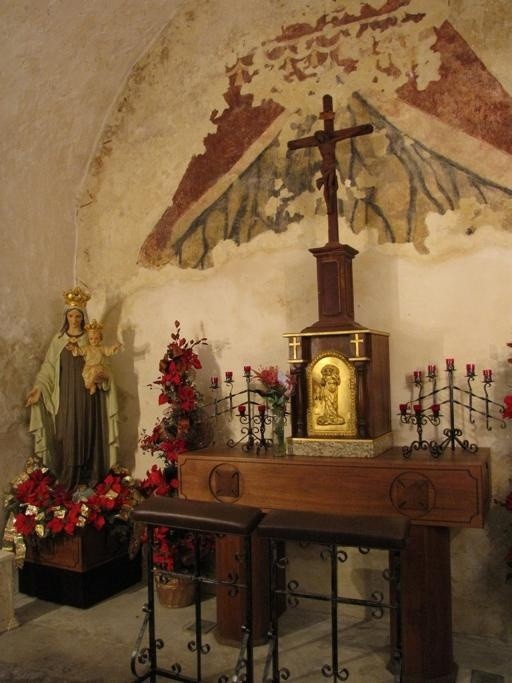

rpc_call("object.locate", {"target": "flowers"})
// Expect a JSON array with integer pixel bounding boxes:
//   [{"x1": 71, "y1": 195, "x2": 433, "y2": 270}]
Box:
[
  {"x1": 126, "y1": 319, "x2": 213, "y2": 573},
  {"x1": 1, "y1": 460, "x2": 137, "y2": 540}
]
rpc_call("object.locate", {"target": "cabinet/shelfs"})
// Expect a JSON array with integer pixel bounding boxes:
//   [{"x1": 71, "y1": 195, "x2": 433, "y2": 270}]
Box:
[{"x1": 176, "y1": 440, "x2": 492, "y2": 683}]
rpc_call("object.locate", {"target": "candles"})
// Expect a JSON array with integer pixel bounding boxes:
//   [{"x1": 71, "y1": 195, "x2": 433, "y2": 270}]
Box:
[
  {"x1": 399, "y1": 357, "x2": 492, "y2": 417},
  {"x1": 211, "y1": 365, "x2": 266, "y2": 416}
]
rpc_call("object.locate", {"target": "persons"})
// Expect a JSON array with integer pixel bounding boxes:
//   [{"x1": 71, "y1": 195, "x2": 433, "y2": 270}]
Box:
[
  {"x1": 312, "y1": 366, "x2": 340, "y2": 422},
  {"x1": 291, "y1": 123, "x2": 370, "y2": 216},
  {"x1": 65, "y1": 331, "x2": 122, "y2": 396},
  {"x1": 24, "y1": 308, "x2": 108, "y2": 496}
]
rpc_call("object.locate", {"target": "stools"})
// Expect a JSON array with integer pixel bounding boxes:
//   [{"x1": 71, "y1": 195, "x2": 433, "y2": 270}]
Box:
[
  {"x1": 255, "y1": 510, "x2": 412, "y2": 683},
  {"x1": 131, "y1": 498, "x2": 264, "y2": 683}
]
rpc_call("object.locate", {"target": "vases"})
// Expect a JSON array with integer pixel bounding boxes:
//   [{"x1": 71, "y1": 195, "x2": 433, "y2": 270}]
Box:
[{"x1": 151, "y1": 568, "x2": 197, "y2": 609}]
